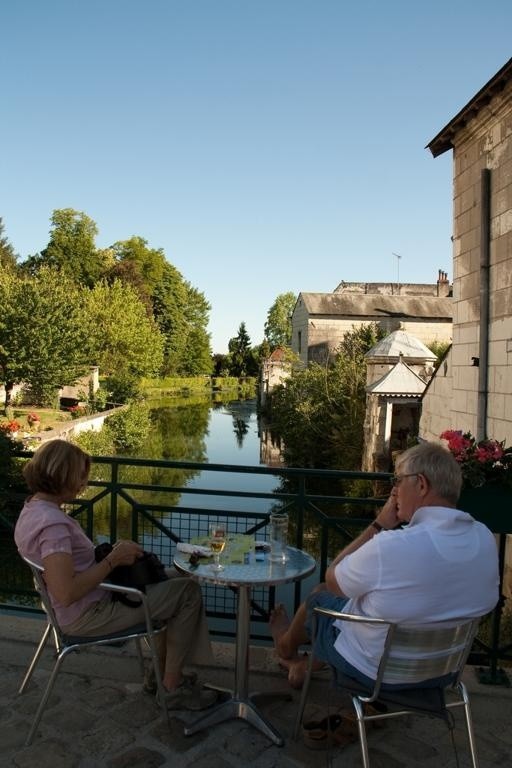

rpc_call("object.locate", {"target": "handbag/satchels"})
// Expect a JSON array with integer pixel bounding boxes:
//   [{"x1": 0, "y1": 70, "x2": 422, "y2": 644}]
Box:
[{"x1": 95, "y1": 543, "x2": 169, "y2": 587}]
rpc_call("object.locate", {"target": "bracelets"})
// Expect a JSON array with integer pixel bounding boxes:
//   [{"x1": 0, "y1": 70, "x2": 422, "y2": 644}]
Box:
[
  {"x1": 104, "y1": 558, "x2": 113, "y2": 573},
  {"x1": 370, "y1": 521, "x2": 389, "y2": 533}
]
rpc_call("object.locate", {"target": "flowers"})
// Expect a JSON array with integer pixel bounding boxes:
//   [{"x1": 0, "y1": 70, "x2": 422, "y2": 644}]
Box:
[
  {"x1": 0, "y1": 413, "x2": 40, "y2": 432},
  {"x1": 405, "y1": 429, "x2": 512, "y2": 491}
]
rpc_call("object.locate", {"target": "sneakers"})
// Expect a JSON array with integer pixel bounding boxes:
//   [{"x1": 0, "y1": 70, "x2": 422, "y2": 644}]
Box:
[
  {"x1": 156, "y1": 678, "x2": 216, "y2": 710},
  {"x1": 363, "y1": 701, "x2": 388, "y2": 728},
  {"x1": 303, "y1": 714, "x2": 369, "y2": 750},
  {"x1": 142, "y1": 667, "x2": 197, "y2": 696}
]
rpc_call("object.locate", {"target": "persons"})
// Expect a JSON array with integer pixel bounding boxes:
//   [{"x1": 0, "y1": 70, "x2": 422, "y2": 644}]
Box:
[
  {"x1": 266, "y1": 440, "x2": 502, "y2": 695},
  {"x1": 14, "y1": 439, "x2": 234, "y2": 713}
]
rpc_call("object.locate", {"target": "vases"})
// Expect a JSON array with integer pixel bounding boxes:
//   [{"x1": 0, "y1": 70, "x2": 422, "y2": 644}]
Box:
[{"x1": 4, "y1": 431, "x2": 18, "y2": 440}]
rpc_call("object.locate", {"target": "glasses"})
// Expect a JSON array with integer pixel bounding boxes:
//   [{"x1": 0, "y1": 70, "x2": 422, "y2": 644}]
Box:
[{"x1": 390, "y1": 472, "x2": 430, "y2": 488}]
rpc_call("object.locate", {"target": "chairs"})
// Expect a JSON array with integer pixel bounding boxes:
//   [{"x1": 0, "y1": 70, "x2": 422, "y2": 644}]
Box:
[
  {"x1": 292, "y1": 607, "x2": 480, "y2": 768},
  {"x1": 16, "y1": 549, "x2": 172, "y2": 746}
]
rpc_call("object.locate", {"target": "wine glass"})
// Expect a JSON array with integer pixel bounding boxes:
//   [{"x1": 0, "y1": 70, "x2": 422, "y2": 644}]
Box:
[
  {"x1": 213, "y1": 525, "x2": 226, "y2": 538},
  {"x1": 209, "y1": 538, "x2": 226, "y2": 571}
]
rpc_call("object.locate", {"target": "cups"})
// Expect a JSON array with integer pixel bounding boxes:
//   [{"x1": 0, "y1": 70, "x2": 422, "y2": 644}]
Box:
[{"x1": 268, "y1": 514, "x2": 290, "y2": 563}]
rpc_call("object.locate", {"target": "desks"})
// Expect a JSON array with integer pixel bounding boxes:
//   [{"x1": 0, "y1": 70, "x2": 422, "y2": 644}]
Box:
[{"x1": 172, "y1": 541, "x2": 317, "y2": 748}]
